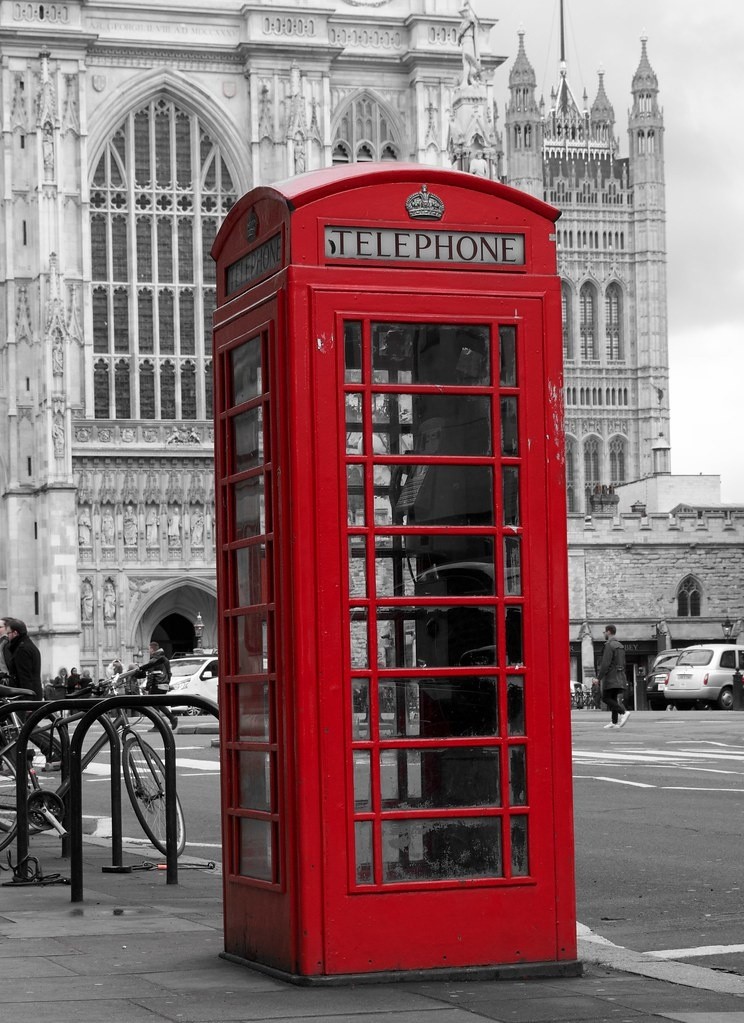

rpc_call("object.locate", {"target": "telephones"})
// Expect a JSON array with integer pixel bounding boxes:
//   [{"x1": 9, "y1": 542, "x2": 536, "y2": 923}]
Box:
[{"x1": 387, "y1": 418, "x2": 482, "y2": 554}]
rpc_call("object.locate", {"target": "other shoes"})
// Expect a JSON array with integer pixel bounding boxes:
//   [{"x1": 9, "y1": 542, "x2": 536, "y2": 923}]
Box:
[
  {"x1": 619, "y1": 711, "x2": 632, "y2": 727},
  {"x1": 604, "y1": 722, "x2": 618, "y2": 729},
  {"x1": 148, "y1": 726, "x2": 160, "y2": 732},
  {"x1": 171, "y1": 718, "x2": 179, "y2": 730}
]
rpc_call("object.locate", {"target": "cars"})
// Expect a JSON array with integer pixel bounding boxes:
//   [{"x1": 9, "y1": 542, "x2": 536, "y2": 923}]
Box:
[
  {"x1": 664, "y1": 644, "x2": 744, "y2": 710},
  {"x1": 645, "y1": 646, "x2": 709, "y2": 711},
  {"x1": 138, "y1": 655, "x2": 268, "y2": 718}
]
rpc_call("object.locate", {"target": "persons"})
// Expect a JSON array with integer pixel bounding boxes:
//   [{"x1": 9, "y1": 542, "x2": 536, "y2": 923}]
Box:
[
  {"x1": 3, "y1": 620, "x2": 73, "y2": 772},
  {"x1": 592, "y1": 625, "x2": 630, "y2": 729},
  {"x1": 469, "y1": 150, "x2": 489, "y2": 178},
  {"x1": 570, "y1": 676, "x2": 601, "y2": 709},
  {"x1": 43, "y1": 119, "x2": 69, "y2": 460},
  {"x1": 0, "y1": 617, "x2": 19, "y2": 775},
  {"x1": 41, "y1": 672, "x2": 108, "y2": 714},
  {"x1": 295, "y1": 141, "x2": 307, "y2": 174},
  {"x1": 107, "y1": 659, "x2": 142, "y2": 717},
  {"x1": 53, "y1": 668, "x2": 69, "y2": 718},
  {"x1": 66, "y1": 667, "x2": 81, "y2": 715},
  {"x1": 77, "y1": 505, "x2": 221, "y2": 625},
  {"x1": 146, "y1": 642, "x2": 179, "y2": 732}
]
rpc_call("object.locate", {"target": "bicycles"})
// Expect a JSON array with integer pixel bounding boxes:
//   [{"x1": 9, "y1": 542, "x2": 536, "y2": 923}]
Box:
[{"x1": 0, "y1": 655, "x2": 187, "y2": 858}]
